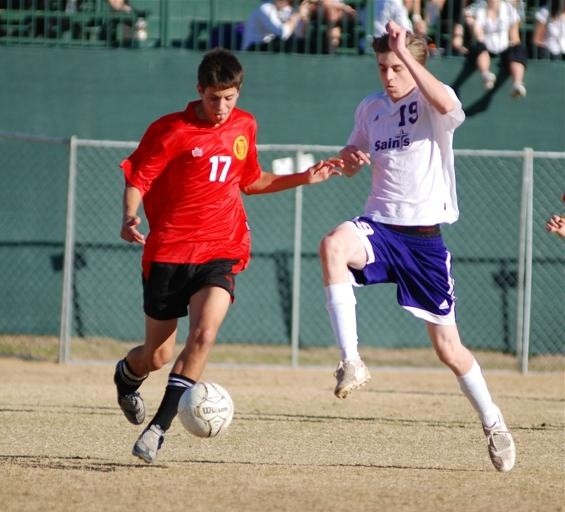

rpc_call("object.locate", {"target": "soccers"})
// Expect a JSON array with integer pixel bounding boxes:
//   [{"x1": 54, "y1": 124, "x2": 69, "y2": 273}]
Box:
[{"x1": 177, "y1": 383, "x2": 234, "y2": 438}]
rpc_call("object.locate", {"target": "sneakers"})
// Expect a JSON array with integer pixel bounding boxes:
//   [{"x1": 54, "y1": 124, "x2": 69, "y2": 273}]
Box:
[
  {"x1": 478, "y1": 403, "x2": 516, "y2": 472},
  {"x1": 117, "y1": 388, "x2": 145, "y2": 424},
  {"x1": 133, "y1": 425, "x2": 163, "y2": 463},
  {"x1": 332, "y1": 358, "x2": 371, "y2": 398}
]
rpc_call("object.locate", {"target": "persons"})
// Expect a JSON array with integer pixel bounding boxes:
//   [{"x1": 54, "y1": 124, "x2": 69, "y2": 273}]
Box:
[
  {"x1": 546, "y1": 194, "x2": 565, "y2": 238},
  {"x1": 114, "y1": 48, "x2": 344, "y2": 464},
  {"x1": 106, "y1": 0, "x2": 147, "y2": 47},
  {"x1": 319, "y1": 19, "x2": 516, "y2": 471}
]
